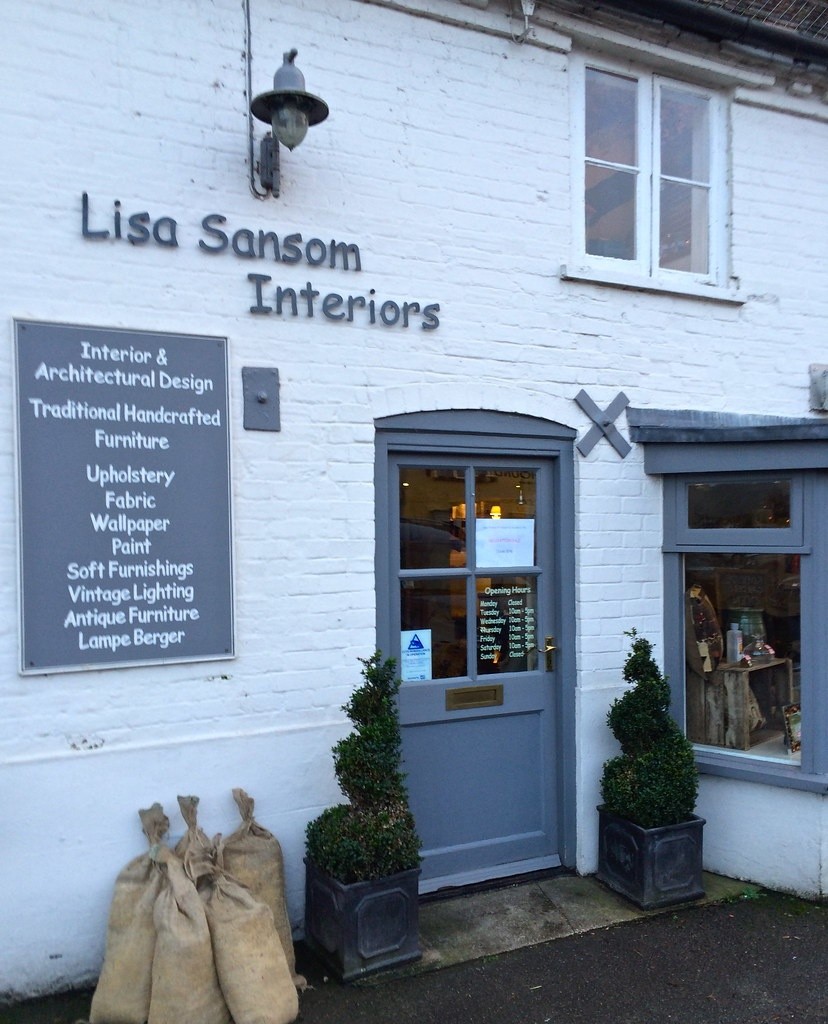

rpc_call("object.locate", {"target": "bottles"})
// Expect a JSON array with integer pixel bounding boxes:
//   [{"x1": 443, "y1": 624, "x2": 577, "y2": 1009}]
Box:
[{"x1": 727, "y1": 623, "x2": 743, "y2": 663}]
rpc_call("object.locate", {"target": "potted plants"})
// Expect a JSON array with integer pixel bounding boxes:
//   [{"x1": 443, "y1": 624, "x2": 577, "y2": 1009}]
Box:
[
  {"x1": 302, "y1": 649, "x2": 427, "y2": 983},
  {"x1": 596, "y1": 626, "x2": 707, "y2": 911}
]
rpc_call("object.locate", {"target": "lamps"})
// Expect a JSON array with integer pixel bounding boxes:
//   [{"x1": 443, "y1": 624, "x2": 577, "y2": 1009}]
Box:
[{"x1": 247, "y1": 48, "x2": 329, "y2": 198}]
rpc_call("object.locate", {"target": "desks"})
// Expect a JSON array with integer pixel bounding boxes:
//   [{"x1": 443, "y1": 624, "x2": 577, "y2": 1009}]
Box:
[{"x1": 706, "y1": 658, "x2": 794, "y2": 750}]
